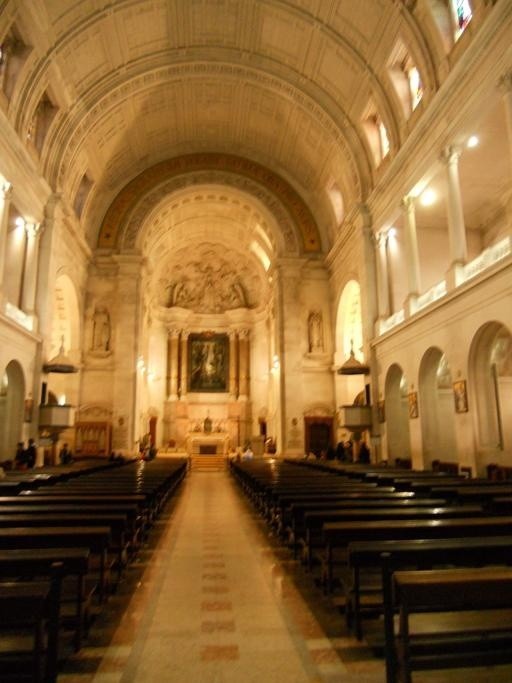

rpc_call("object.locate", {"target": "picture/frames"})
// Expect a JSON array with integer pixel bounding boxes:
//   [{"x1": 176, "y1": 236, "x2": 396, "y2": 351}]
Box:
[
  {"x1": 376, "y1": 400, "x2": 385, "y2": 424},
  {"x1": 453, "y1": 379, "x2": 468, "y2": 413},
  {"x1": 407, "y1": 392, "x2": 418, "y2": 419}
]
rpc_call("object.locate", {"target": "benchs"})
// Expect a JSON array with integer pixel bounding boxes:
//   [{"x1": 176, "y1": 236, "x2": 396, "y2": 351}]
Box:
[
  {"x1": 226, "y1": 458, "x2": 512, "y2": 683},
  {"x1": 0, "y1": 458, "x2": 189, "y2": 683}
]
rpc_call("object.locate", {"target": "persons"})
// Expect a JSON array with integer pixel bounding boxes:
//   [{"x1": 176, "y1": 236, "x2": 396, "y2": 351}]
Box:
[
  {"x1": 311, "y1": 441, "x2": 370, "y2": 465},
  {"x1": 25, "y1": 439, "x2": 38, "y2": 470},
  {"x1": 227, "y1": 446, "x2": 254, "y2": 464},
  {"x1": 14, "y1": 442, "x2": 25, "y2": 470},
  {"x1": 58, "y1": 443, "x2": 68, "y2": 464}
]
